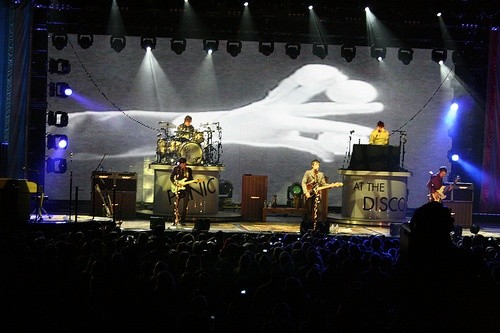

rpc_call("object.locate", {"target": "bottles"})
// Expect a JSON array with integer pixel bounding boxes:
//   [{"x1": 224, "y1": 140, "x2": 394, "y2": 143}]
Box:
[{"x1": 332, "y1": 224, "x2": 339, "y2": 234}]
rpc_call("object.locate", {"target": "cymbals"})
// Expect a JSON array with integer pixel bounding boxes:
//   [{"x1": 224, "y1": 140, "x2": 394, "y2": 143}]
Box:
[
  {"x1": 174, "y1": 130, "x2": 193, "y2": 134},
  {"x1": 158, "y1": 121, "x2": 176, "y2": 128},
  {"x1": 200, "y1": 122, "x2": 217, "y2": 126},
  {"x1": 200, "y1": 129, "x2": 215, "y2": 132}
]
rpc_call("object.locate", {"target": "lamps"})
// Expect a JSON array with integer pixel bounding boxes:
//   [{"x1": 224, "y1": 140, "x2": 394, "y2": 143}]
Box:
[
  {"x1": 48, "y1": 57, "x2": 72, "y2": 76},
  {"x1": 45, "y1": 157, "x2": 67, "y2": 174},
  {"x1": 287, "y1": 182, "x2": 303, "y2": 200},
  {"x1": 46, "y1": 111, "x2": 69, "y2": 128},
  {"x1": 219, "y1": 179, "x2": 234, "y2": 198},
  {"x1": 447, "y1": 149, "x2": 459, "y2": 163},
  {"x1": 52, "y1": 33, "x2": 467, "y2": 65},
  {"x1": 46, "y1": 133, "x2": 68, "y2": 151},
  {"x1": 32, "y1": 23, "x2": 48, "y2": 31},
  {"x1": 47, "y1": 81, "x2": 72, "y2": 99}
]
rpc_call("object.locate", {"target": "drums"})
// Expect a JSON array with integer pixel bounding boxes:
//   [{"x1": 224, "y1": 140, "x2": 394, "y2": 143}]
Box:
[
  {"x1": 156, "y1": 139, "x2": 168, "y2": 154},
  {"x1": 190, "y1": 131, "x2": 204, "y2": 144},
  {"x1": 165, "y1": 136, "x2": 183, "y2": 154},
  {"x1": 178, "y1": 133, "x2": 190, "y2": 140},
  {"x1": 177, "y1": 141, "x2": 203, "y2": 165}
]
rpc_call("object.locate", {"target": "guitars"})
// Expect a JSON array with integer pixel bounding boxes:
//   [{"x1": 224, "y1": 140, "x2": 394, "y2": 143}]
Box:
[
  {"x1": 170, "y1": 177, "x2": 204, "y2": 194},
  {"x1": 303, "y1": 180, "x2": 344, "y2": 200},
  {"x1": 428, "y1": 175, "x2": 461, "y2": 203}
]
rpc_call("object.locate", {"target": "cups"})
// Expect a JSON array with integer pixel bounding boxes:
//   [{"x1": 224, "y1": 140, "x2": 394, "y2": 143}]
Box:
[{"x1": 264, "y1": 200, "x2": 272, "y2": 207}]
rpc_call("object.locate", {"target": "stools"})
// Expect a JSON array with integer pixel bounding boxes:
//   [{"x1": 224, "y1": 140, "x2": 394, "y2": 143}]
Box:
[{"x1": 30, "y1": 195, "x2": 52, "y2": 220}]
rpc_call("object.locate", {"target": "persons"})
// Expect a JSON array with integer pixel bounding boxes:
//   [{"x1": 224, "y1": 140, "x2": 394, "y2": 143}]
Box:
[
  {"x1": 369, "y1": 121, "x2": 389, "y2": 145},
  {"x1": 427, "y1": 167, "x2": 453, "y2": 204},
  {"x1": 301, "y1": 159, "x2": 335, "y2": 223},
  {"x1": 0, "y1": 230, "x2": 500, "y2": 333},
  {"x1": 176, "y1": 116, "x2": 194, "y2": 140},
  {"x1": 170, "y1": 157, "x2": 200, "y2": 226}
]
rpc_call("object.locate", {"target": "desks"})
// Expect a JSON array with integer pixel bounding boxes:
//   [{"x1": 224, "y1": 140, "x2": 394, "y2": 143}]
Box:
[
  {"x1": 149, "y1": 165, "x2": 225, "y2": 216},
  {"x1": 337, "y1": 169, "x2": 412, "y2": 220}
]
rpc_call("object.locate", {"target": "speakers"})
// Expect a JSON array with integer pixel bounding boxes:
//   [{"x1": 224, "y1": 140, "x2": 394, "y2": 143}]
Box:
[
  {"x1": 390, "y1": 223, "x2": 403, "y2": 236},
  {"x1": 300, "y1": 221, "x2": 329, "y2": 233},
  {"x1": 92, "y1": 170, "x2": 138, "y2": 191},
  {"x1": 194, "y1": 218, "x2": 210, "y2": 230},
  {"x1": 150, "y1": 217, "x2": 165, "y2": 229},
  {"x1": 442, "y1": 182, "x2": 474, "y2": 203}
]
